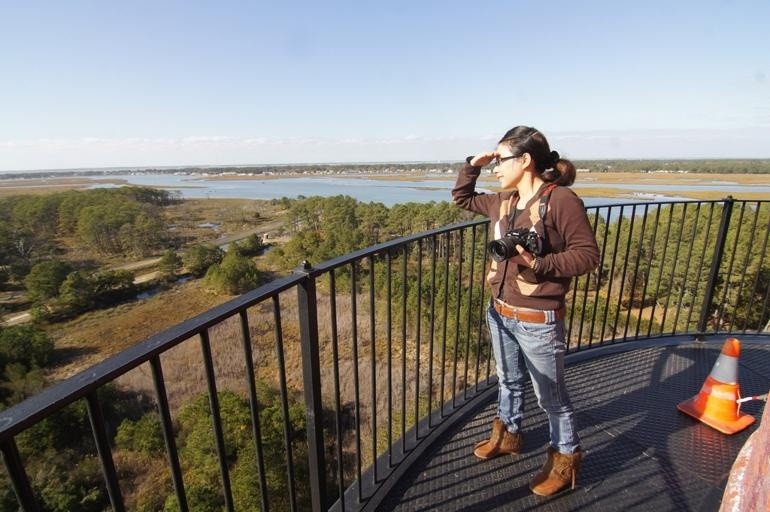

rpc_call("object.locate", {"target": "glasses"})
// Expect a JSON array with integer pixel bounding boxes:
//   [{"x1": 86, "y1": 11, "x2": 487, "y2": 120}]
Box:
[{"x1": 494, "y1": 154, "x2": 519, "y2": 166}]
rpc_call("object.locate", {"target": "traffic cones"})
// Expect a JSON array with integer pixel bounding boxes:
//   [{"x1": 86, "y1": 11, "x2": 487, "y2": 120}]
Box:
[{"x1": 673, "y1": 336, "x2": 759, "y2": 438}]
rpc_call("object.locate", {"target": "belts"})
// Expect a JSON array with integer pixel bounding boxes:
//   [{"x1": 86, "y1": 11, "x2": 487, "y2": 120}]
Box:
[{"x1": 492, "y1": 298, "x2": 566, "y2": 323}]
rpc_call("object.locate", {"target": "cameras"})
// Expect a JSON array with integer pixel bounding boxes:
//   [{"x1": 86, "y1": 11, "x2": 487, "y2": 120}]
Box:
[{"x1": 488, "y1": 226, "x2": 539, "y2": 263}]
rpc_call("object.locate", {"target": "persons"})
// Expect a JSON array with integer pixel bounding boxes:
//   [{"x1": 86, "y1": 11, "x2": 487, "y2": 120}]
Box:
[{"x1": 450, "y1": 125, "x2": 602, "y2": 498}]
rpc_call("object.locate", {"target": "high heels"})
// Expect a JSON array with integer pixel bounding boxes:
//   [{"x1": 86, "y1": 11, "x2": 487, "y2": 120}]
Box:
[
  {"x1": 472, "y1": 417, "x2": 523, "y2": 460},
  {"x1": 528, "y1": 446, "x2": 582, "y2": 497}
]
